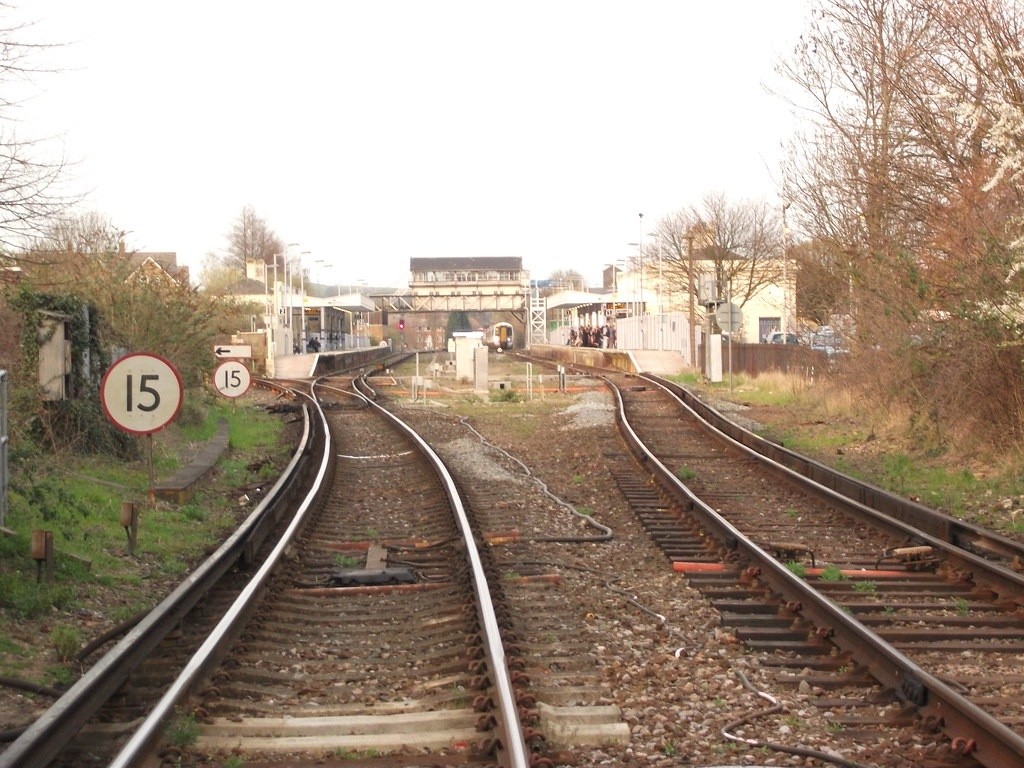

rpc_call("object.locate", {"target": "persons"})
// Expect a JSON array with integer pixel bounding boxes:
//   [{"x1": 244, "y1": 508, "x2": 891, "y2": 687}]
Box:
[
  {"x1": 294, "y1": 337, "x2": 321, "y2": 355},
  {"x1": 566, "y1": 325, "x2": 616, "y2": 349}
]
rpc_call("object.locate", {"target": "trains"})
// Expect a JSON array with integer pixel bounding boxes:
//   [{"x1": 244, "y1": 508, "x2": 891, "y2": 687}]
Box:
[{"x1": 483, "y1": 322, "x2": 514, "y2": 350}]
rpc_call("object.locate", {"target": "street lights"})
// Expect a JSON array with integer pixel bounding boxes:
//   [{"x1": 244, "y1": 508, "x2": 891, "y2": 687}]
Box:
[
  {"x1": 638, "y1": 213, "x2": 646, "y2": 325},
  {"x1": 603, "y1": 241, "x2": 642, "y2": 320},
  {"x1": 781, "y1": 203, "x2": 794, "y2": 344},
  {"x1": 648, "y1": 232, "x2": 663, "y2": 307},
  {"x1": 264, "y1": 242, "x2": 333, "y2": 352}
]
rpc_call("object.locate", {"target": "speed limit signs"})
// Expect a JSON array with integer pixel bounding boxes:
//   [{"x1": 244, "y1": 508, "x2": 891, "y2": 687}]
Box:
[
  {"x1": 101, "y1": 354, "x2": 184, "y2": 437},
  {"x1": 213, "y1": 358, "x2": 252, "y2": 401}
]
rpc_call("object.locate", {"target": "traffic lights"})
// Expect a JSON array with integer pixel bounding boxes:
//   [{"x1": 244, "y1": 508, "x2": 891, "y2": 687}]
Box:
[{"x1": 399, "y1": 319, "x2": 406, "y2": 330}]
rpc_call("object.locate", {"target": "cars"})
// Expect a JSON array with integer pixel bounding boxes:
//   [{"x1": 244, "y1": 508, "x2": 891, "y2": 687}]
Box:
[{"x1": 762, "y1": 325, "x2": 846, "y2": 354}]
